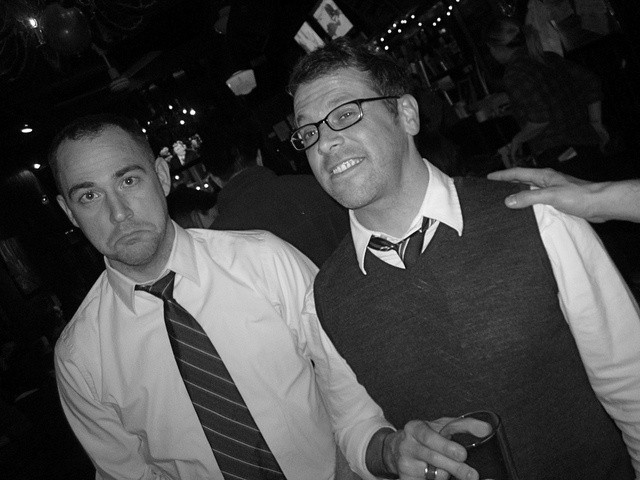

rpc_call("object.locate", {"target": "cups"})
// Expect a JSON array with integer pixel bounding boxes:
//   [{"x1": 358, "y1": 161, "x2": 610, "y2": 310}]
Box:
[{"x1": 439, "y1": 410, "x2": 519, "y2": 480}]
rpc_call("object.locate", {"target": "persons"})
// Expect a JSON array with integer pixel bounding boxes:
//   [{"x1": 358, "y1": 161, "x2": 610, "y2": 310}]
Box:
[
  {"x1": 196, "y1": 110, "x2": 349, "y2": 269},
  {"x1": 484, "y1": 19, "x2": 610, "y2": 166},
  {"x1": 486, "y1": 163, "x2": 640, "y2": 227},
  {"x1": 283, "y1": 41, "x2": 640, "y2": 478},
  {"x1": 46, "y1": 110, "x2": 336, "y2": 480}
]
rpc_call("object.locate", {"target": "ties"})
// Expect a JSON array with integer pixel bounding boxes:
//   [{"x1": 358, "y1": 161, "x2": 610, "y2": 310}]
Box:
[
  {"x1": 135, "y1": 274, "x2": 284, "y2": 479},
  {"x1": 368, "y1": 214, "x2": 437, "y2": 269}
]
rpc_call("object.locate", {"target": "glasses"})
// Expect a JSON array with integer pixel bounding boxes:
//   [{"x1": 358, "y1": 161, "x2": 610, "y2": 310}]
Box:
[{"x1": 289, "y1": 95, "x2": 398, "y2": 152}]
